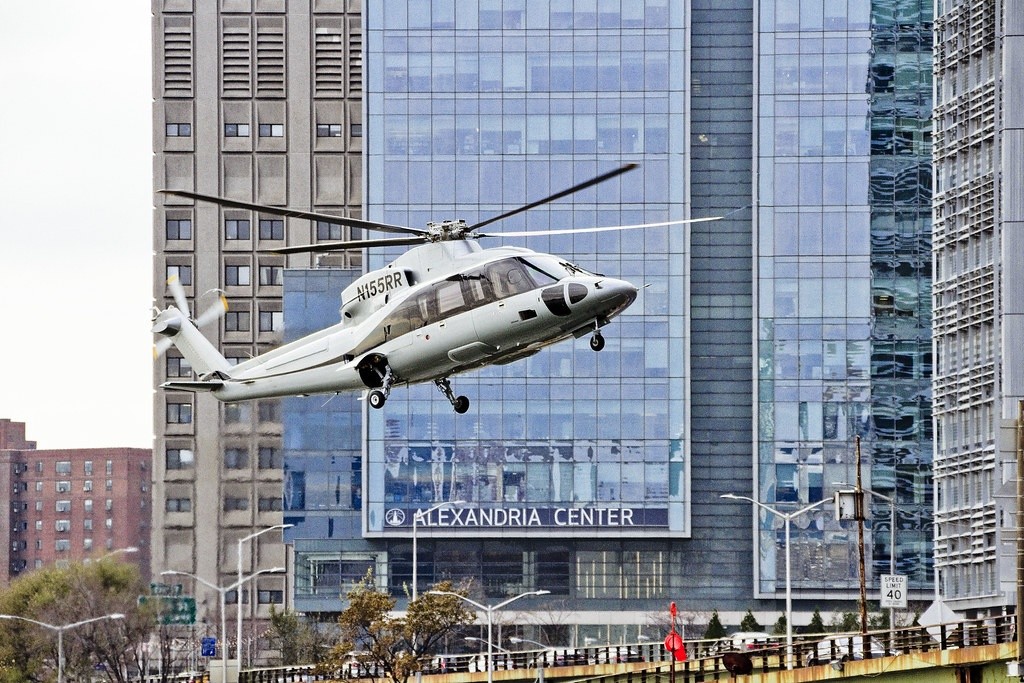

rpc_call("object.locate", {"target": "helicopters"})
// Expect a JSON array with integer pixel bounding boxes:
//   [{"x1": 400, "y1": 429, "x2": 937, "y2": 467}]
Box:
[{"x1": 150, "y1": 162, "x2": 727, "y2": 415}]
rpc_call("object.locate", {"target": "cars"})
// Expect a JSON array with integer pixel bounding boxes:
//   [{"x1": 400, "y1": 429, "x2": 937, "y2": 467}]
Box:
[
  {"x1": 805, "y1": 637, "x2": 900, "y2": 666},
  {"x1": 333, "y1": 651, "x2": 385, "y2": 678},
  {"x1": 529, "y1": 645, "x2": 586, "y2": 667},
  {"x1": 727, "y1": 631, "x2": 779, "y2": 652},
  {"x1": 469, "y1": 651, "x2": 512, "y2": 673},
  {"x1": 588, "y1": 648, "x2": 645, "y2": 665}
]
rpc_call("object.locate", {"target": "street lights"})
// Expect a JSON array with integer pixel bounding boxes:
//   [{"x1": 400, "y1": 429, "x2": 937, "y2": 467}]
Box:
[
  {"x1": 97, "y1": 548, "x2": 138, "y2": 562},
  {"x1": 426, "y1": 590, "x2": 551, "y2": 683},
  {"x1": 236, "y1": 524, "x2": 294, "y2": 674},
  {"x1": 412, "y1": 501, "x2": 467, "y2": 658},
  {"x1": 831, "y1": 481, "x2": 896, "y2": 656},
  {"x1": 510, "y1": 638, "x2": 547, "y2": 648},
  {"x1": 464, "y1": 637, "x2": 510, "y2": 658},
  {"x1": 719, "y1": 494, "x2": 835, "y2": 670},
  {"x1": 161, "y1": 567, "x2": 285, "y2": 683},
  {"x1": 1, "y1": 614, "x2": 126, "y2": 683}
]
